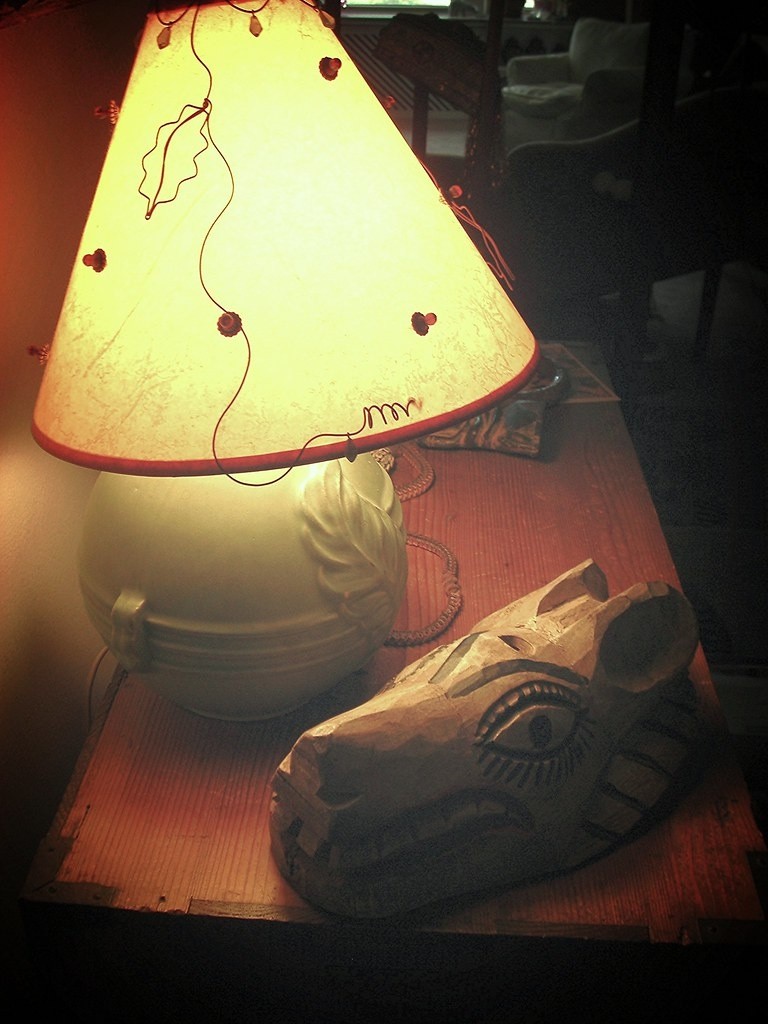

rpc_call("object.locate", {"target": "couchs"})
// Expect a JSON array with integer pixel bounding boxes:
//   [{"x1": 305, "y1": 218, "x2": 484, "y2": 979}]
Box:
[{"x1": 501, "y1": 17, "x2": 652, "y2": 146}]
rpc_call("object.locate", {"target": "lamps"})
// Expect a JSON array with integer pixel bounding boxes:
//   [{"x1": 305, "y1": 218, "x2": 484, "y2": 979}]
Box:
[{"x1": 31, "y1": 0, "x2": 539, "y2": 722}]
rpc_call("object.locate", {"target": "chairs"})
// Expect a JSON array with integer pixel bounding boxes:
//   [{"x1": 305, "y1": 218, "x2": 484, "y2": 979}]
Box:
[{"x1": 387, "y1": 0, "x2": 768, "y2": 380}]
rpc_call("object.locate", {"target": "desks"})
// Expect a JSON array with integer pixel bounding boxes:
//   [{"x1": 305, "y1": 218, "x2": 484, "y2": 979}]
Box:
[{"x1": 24, "y1": 337, "x2": 768, "y2": 1024}]
